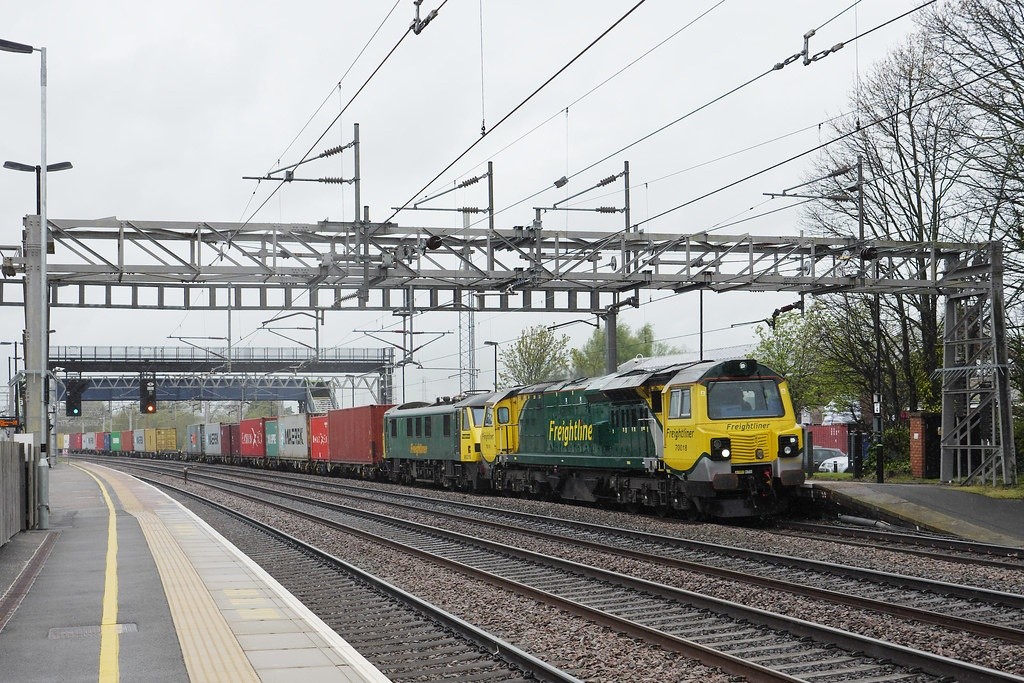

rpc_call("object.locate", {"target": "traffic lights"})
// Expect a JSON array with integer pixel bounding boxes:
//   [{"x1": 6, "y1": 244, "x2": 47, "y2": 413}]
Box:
[
  {"x1": 146, "y1": 393, "x2": 154, "y2": 412},
  {"x1": 71, "y1": 390, "x2": 79, "y2": 414}
]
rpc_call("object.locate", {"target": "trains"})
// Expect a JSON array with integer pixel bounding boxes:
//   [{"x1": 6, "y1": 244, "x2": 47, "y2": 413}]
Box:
[{"x1": 55, "y1": 356, "x2": 806, "y2": 520}]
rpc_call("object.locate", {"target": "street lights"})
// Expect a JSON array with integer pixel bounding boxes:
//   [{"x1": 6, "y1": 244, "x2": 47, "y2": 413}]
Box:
[
  {"x1": 0, "y1": 342, "x2": 24, "y2": 431},
  {"x1": 0, "y1": 39, "x2": 52, "y2": 531},
  {"x1": 2, "y1": 160, "x2": 73, "y2": 216}
]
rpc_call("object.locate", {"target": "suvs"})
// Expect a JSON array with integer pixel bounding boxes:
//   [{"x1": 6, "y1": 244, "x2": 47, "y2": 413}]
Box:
[{"x1": 813, "y1": 447, "x2": 846, "y2": 472}]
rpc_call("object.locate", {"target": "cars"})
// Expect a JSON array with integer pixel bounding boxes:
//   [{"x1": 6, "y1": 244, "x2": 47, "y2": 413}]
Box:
[{"x1": 818, "y1": 451, "x2": 849, "y2": 473}]
